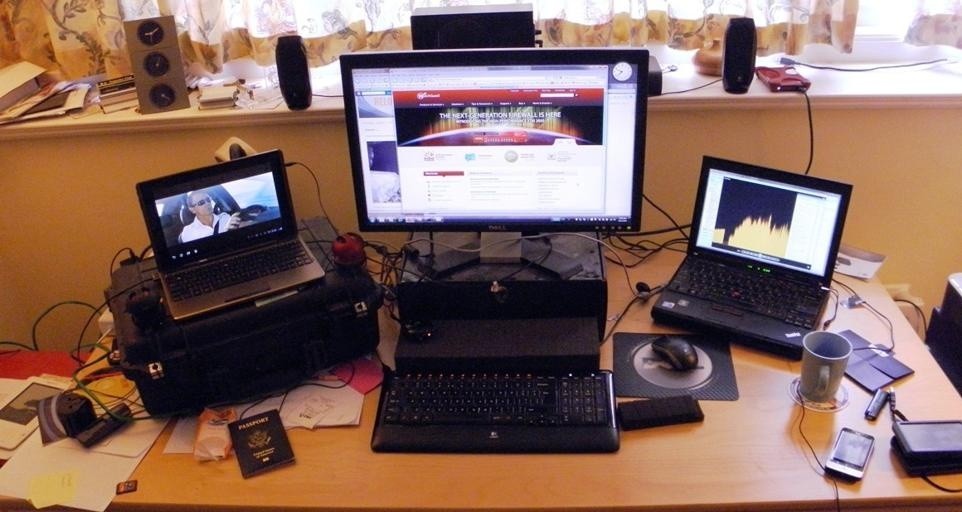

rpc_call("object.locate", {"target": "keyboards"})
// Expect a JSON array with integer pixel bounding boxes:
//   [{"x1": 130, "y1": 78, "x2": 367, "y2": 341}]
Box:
[{"x1": 369, "y1": 368, "x2": 620, "y2": 455}]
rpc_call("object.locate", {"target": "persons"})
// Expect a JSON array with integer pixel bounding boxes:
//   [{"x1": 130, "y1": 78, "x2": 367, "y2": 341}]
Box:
[
  {"x1": 180, "y1": 190, "x2": 241, "y2": 244},
  {"x1": 0, "y1": 59, "x2": 138, "y2": 125}
]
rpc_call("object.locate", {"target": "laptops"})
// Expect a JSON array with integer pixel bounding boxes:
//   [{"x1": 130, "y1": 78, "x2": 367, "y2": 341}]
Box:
[
  {"x1": 649, "y1": 155, "x2": 854, "y2": 362},
  {"x1": 135, "y1": 145, "x2": 325, "y2": 321}
]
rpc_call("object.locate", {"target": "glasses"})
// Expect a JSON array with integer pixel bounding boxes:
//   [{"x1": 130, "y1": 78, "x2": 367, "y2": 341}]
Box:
[{"x1": 192, "y1": 197, "x2": 211, "y2": 207}]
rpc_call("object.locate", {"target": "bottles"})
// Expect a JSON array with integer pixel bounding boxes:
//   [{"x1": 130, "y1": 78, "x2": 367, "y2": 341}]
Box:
[{"x1": 694, "y1": 39, "x2": 722, "y2": 75}]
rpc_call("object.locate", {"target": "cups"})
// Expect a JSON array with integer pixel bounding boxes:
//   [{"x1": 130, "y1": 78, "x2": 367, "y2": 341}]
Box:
[{"x1": 800, "y1": 328, "x2": 852, "y2": 402}]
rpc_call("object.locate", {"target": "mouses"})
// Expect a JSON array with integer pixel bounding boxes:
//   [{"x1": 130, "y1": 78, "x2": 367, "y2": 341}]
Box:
[{"x1": 653, "y1": 335, "x2": 697, "y2": 372}]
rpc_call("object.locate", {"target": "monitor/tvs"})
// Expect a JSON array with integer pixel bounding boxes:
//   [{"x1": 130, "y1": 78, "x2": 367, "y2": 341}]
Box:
[{"x1": 339, "y1": 48, "x2": 650, "y2": 280}]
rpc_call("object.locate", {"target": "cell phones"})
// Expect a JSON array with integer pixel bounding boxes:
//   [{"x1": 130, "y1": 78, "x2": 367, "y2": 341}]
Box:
[{"x1": 825, "y1": 427, "x2": 875, "y2": 481}]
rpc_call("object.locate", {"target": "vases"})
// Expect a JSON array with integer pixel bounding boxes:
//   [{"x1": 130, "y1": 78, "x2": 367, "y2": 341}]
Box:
[{"x1": 693, "y1": 36, "x2": 725, "y2": 77}]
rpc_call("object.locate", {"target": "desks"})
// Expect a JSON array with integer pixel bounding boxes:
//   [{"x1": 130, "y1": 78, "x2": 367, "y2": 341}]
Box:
[{"x1": 2, "y1": 241, "x2": 962, "y2": 512}]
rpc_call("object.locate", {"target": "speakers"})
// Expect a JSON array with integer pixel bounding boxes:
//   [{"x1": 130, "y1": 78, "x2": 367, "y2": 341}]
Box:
[
  {"x1": 724, "y1": 17, "x2": 756, "y2": 93},
  {"x1": 276, "y1": 35, "x2": 312, "y2": 109}
]
